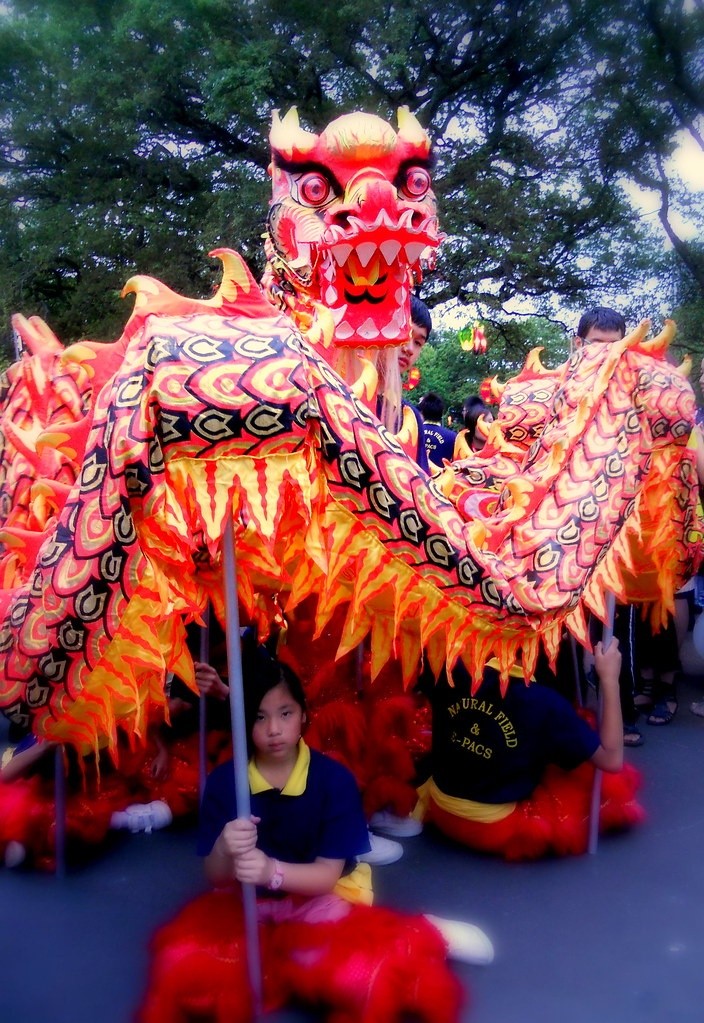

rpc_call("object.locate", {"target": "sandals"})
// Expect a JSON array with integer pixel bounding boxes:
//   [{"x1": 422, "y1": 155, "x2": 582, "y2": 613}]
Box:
[
  {"x1": 622, "y1": 726, "x2": 644, "y2": 747},
  {"x1": 634, "y1": 692, "x2": 654, "y2": 706},
  {"x1": 649, "y1": 701, "x2": 676, "y2": 723}
]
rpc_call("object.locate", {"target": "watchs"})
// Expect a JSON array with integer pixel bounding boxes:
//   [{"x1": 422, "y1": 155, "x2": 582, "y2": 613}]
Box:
[{"x1": 265, "y1": 858, "x2": 285, "y2": 892}]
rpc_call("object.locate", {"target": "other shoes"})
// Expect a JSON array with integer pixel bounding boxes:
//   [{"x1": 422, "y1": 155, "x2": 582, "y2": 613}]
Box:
[{"x1": 690, "y1": 701, "x2": 704, "y2": 715}]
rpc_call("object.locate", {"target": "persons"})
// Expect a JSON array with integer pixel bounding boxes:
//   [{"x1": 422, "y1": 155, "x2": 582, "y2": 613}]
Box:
[{"x1": 0, "y1": 292, "x2": 704, "y2": 1023}]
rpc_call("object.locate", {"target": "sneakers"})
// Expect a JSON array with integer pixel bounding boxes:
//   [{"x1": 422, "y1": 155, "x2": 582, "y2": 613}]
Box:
[
  {"x1": 425, "y1": 914, "x2": 495, "y2": 965},
  {"x1": 125, "y1": 800, "x2": 173, "y2": 834},
  {"x1": 354, "y1": 830, "x2": 404, "y2": 865},
  {"x1": 370, "y1": 811, "x2": 423, "y2": 837},
  {"x1": 6, "y1": 842, "x2": 26, "y2": 867}
]
